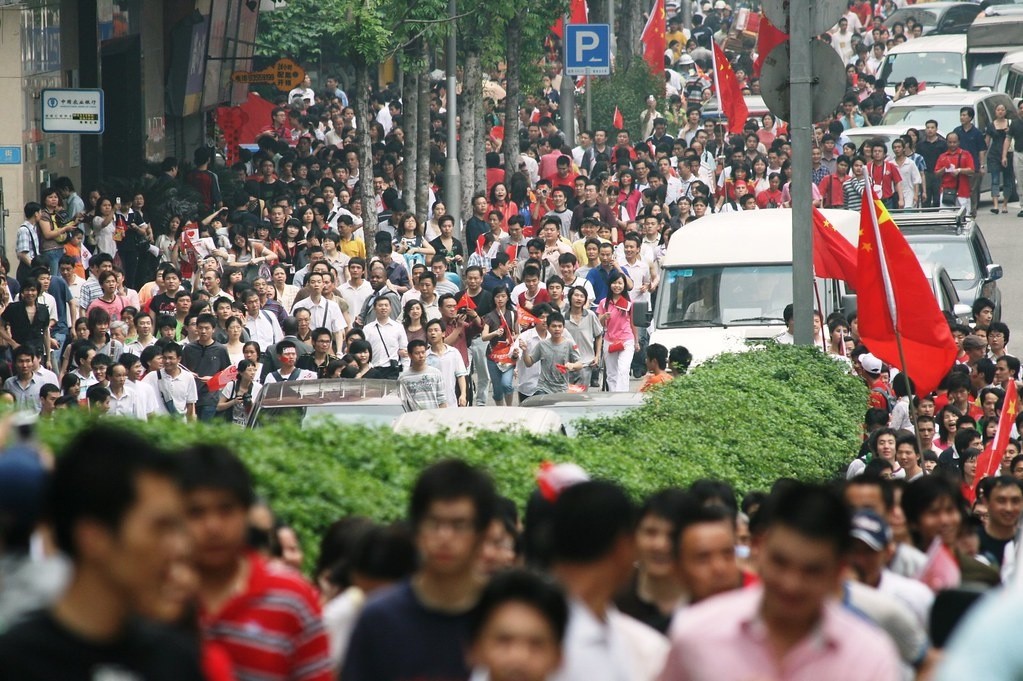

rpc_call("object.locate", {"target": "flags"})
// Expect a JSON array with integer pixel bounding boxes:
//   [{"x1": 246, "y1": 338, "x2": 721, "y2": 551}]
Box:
[
  {"x1": 550, "y1": 0, "x2": 588, "y2": 39},
  {"x1": 752, "y1": 9, "x2": 790, "y2": 76},
  {"x1": 968, "y1": 381, "x2": 1020, "y2": 505},
  {"x1": 206, "y1": 363, "x2": 238, "y2": 392},
  {"x1": 639, "y1": 0, "x2": 666, "y2": 79},
  {"x1": 557, "y1": 364, "x2": 567, "y2": 373},
  {"x1": 858, "y1": 184, "x2": 957, "y2": 399},
  {"x1": 695, "y1": 63, "x2": 715, "y2": 92},
  {"x1": 505, "y1": 245, "x2": 516, "y2": 263},
  {"x1": 475, "y1": 235, "x2": 486, "y2": 254},
  {"x1": 813, "y1": 205, "x2": 857, "y2": 293},
  {"x1": 517, "y1": 304, "x2": 542, "y2": 324},
  {"x1": 613, "y1": 106, "x2": 624, "y2": 129},
  {"x1": 491, "y1": 343, "x2": 516, "y2": 364},
  {"x1": 711, "y1": 35, "x2": 749, "y2": 134},
  {"x1": 456, "y1": 293, "x2": 478, "y2": 310}
]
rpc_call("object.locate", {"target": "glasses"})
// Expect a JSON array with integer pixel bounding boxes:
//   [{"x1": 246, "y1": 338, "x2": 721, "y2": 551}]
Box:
[
  {"x1": 276, "y1": 113, "x2": 286, "y2": 118},
  {"x1": 882, "y1": 473, "x2": 896, "y2": 480}
]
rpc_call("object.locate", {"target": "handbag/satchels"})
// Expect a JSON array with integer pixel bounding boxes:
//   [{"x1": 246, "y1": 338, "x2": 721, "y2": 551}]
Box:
[
  {"x1": 942, "y1": 188, "x2": 957, "y2": 205},
  {"x1": 211, "y1": 380, "x2": 235, "y2": 426},
  {"x1": 1007, "y1": 119, "x2": 1015, "y2": 152},
  {"x1": 390, "y1": 359, "x2": 398, "y2": 369}
]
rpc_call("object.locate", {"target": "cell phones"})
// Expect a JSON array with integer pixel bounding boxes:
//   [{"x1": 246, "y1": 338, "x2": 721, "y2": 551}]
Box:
[
  {"x1": 116, "y1": 197, "x2": 121, "y2": 204},
  {"x1": 74, "y1": 221, "x2": 80, "y2": 226},
  {"x1": 649, "y1": 95, "x2": 653, "y2": 101}
]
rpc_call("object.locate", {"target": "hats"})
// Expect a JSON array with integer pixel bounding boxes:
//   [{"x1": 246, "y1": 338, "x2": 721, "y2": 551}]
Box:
[
  {"x1": 858, "y1": 353, "x2": 882, "y2": 374},
  {"x1": 702, "y1": 3, "x2": 711, "y2": 11},
  {"x1": 849, "y1": 510, "x2": 891, "y2": 552},
  {"x1": 963, "y1": 335, "x2": 988, "y2": 349},
  {"x1": 714, "y1": 0, "x2": 726, "y2": 9},
  {"x1": 734, "y1": 180, "x2": 747, "y2": 190},
  {"x1": 584, "y1": 217, "x2": 599, "y2": 226},
  {"x1": 678, "y1": 54, "x2": 694, "y2": 65}
]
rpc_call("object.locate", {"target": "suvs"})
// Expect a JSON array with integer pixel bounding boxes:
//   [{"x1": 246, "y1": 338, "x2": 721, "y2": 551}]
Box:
[{"x1": 887, "y1": 206, "x2": 1003, "y2": 329}]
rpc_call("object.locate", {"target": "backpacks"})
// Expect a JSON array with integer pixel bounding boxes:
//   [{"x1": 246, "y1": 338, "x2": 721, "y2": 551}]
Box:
[{"x1": 43, "y1": 209, "x2": 67, "y2": 243}]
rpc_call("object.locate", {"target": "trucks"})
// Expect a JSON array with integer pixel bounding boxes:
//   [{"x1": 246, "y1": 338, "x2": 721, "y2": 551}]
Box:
[{"x1": 960, "y1": 4, "x2": 1023, "y2": 92}]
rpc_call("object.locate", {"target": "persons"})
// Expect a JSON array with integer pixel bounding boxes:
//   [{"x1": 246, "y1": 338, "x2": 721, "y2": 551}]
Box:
[
  {"x1": 0, "y1": 0, "x2": 1023, "y2": 421},
  {"x1": 0, "y1": 298, "x2": 1023, "y2": 681}
]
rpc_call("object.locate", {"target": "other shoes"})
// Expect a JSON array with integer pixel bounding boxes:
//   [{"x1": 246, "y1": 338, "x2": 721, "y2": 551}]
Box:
[
  {"x1": 990, "y1": 208, "x2": 999, "y2": 214},
  {"x1": 1002, "y1": 206, "x2": 1007, "y2": 213},
  {"x1": 1018, "y1": 211, "x2": 1023, "y2": 217}
]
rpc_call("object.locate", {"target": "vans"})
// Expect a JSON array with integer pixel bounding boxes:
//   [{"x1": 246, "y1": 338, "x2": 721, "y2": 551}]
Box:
[{"x1": 633, "y1": 208, "x2": 860, "y2": 376}]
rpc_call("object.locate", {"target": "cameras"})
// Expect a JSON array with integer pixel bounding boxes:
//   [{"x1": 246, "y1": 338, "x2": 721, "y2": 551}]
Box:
[
  {"x1": 241, "y1": 393, "x2": 252, "y2": 408},
  {"x1": 457, "y1": 307, "x2": 466, "y2": 315}
]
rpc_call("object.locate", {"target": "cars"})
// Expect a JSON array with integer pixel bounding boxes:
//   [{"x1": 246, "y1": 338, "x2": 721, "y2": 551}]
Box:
[
  {"x1": 245, "y1": 378, "x2": 418, "y2": 431},
  {"x1": 390, "y1": 406, "x2": 562, "y2": 434},
  {"x1": 876, "y1": 34, "x2": 967, "y2": 97},
  {"x1": 843, "y1": 265, "x2": 972, "y2": 323},
  {"x1": 880, "y1": 91, "x2": 1018, "y2": 193},
  {"x1": 700, "y1": 95, "x2": 784, "y2": 131},
  {"x1": 993, "y1": 48, "x2": 1023, "y2": 107},
  {"x1": 882, "y1": 3, "x2": 982, "y2": 36},
  {"x1": 518, "y1": 392, "x2": 652, "y2": 438},
  {"x1": 842, "y1": 126, "x2": 925, "y2": 159}
]
